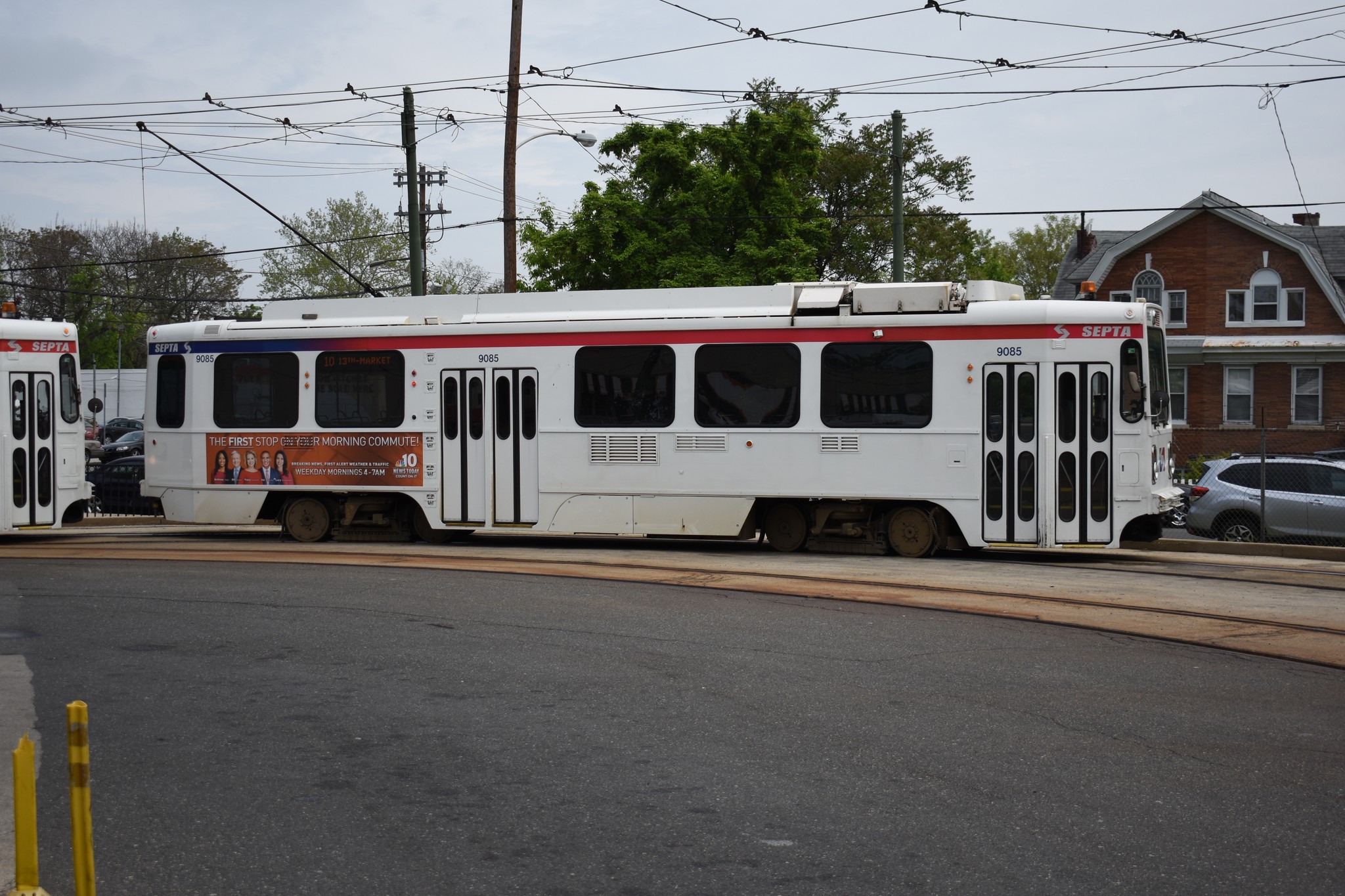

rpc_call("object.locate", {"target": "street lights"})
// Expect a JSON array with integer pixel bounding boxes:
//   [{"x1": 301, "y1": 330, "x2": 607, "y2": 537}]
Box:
[{"x1": 503, "y1": 130, "x2": 597, "y2": 291}]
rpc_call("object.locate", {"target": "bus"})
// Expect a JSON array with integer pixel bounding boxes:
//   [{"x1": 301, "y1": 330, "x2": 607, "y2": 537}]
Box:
[
  {"x1": 0, "y1": 302, "x2": 94, "y2": 538},
  {"x1": 139, "y1": 280, "x2": 1188, "y2": 559}
]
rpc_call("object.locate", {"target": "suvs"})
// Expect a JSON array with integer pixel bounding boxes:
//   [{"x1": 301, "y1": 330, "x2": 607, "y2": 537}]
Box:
[{"x1": 1185, "y1": 451, "x2": 1345, "y2": 546}]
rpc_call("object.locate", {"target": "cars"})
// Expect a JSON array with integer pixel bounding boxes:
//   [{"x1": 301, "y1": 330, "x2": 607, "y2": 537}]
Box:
[
  {"x1": 84, "y1": 438, "x2": 105, "y2": 466},
  {"x1": 99, "y1": 430, "x2": 144, "y2": 463},
  {"x1": 85, "y1": 455, "x2": 165, "y2": 515},
  {"x1": 99, "y1": 419, "x2": 145, "y2": 445},
  {"x1": 107, "y1": 417, "x2": 140, "y2": 424},
  {"x1": 84, "y1": 420, "x2": 100, "y2": 441}
]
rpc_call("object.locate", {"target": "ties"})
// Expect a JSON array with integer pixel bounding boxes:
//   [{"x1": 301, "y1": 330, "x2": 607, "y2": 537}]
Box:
[
  {"x1": 234, "y1": 469, "x2": 238, "y2": 484},
  {"x1": 266, "y1": 470, "x2": 270, "y2": 485}
]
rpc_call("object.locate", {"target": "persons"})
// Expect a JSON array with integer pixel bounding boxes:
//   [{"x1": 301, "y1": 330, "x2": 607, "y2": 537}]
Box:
[
  {"x1": 29, "y1": 398, "x2": 44, "y2": 424},
  {"x1": 13, "y1": 381, "x2": 23, "y2": 426},
  {"x1": 210, "y1": 450, "x2": 294, "y2": 485}
]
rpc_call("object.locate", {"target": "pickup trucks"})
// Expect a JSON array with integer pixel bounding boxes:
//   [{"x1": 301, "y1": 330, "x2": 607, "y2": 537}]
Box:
[{"x1": 1169, "y1": 483, "x2": 1196, "y2": 530}]
